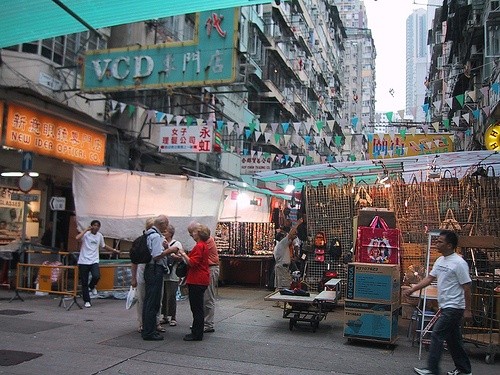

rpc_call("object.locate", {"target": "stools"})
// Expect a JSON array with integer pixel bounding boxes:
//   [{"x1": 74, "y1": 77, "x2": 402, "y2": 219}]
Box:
[
  {"x1": 407, "y1": 310, "x2": 435, "y2": 338},
  {"x1": 324, "y1": 279, "x2": 341, "y2": 300}
]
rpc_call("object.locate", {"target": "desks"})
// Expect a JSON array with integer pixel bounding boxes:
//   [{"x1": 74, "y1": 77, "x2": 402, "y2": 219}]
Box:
[{"x1": 219, "y1": 255, "x2": 274, "y2": 290}]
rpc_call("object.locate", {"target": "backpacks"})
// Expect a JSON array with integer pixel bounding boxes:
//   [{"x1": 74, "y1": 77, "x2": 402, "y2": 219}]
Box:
[
  {"x1": 162, "y1": 239, "x2": 178, "y2": 275},
  {"x1": 130, "y1": 229, "x2": 155, "y2": 264}
]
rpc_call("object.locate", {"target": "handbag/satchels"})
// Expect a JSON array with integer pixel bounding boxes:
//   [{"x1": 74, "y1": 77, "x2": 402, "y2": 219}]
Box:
[
  {"x1": 176, "y1": 261, "x2": 186, "y2": 277},
  {"x1": 258, "y1": 165, "x2": 499, "y2": 297}
]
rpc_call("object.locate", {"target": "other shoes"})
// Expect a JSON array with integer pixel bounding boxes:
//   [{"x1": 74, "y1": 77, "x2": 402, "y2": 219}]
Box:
[
  {"x1": 160, "y1": 318, "x2": 177, "y2": 326},
  {"x1": 137, "y1": 321, "x2": 166, "y2": 333}
]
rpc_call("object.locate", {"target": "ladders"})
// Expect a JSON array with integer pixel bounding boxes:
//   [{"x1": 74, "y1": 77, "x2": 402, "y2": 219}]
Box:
[{"x1": 412, "y1": 232, "x2": 456, "y2": 361}]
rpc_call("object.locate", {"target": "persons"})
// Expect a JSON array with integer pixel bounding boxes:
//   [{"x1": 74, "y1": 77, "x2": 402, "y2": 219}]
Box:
[
  {"x1": 272, "y1": 217, "x2": 303, "y2": 309},
  {"x1": 6, "y1": 208, "x2": 18, "y2": 222},
  {"x1": 75, "y1": 220, "x2": 120, "y2": 308},
  {"x1": 405, "y1": 231, "x2": 472, "y2": 375},
  {"x1": 131, "y1": 216, "x2": 220, "y2": 341},
  {"x1": 0, "y1": 221, "x2": 7, "y2": 229},
  {"x1": 40, "y1": 219, "x2": 64, "y2": 250}
]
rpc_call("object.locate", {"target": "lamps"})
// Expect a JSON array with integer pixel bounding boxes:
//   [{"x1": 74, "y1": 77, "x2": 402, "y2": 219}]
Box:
[{"x1": 284, "y1": 179, "x2": 295, "y2": 194}]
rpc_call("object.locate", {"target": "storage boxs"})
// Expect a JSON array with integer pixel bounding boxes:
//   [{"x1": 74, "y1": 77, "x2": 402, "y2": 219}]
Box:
[
  {"x1": 344, "y1": 298, "x2": 399, "y2": 342},
  {"x1": 347, "y1": 262, "x2": 400, "y2": 303},
  {"x1": 400, "y1": 243, "x2": 443, "y2": 284}
]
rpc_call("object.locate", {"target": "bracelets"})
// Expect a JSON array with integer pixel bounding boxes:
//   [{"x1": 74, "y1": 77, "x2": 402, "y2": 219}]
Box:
[{"x1": 182, "y1": 252, "x2": 186, "y2": 255}]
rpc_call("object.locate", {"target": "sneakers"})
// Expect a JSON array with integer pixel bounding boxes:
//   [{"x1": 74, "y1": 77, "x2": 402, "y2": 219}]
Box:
[
  {"x1": 92, "y1": 288, "x2": 98, "y2": 294},
  {"x1": 143, "y1": 333, "x2": 164, "y2": 340},
  {"x1": 184, "y1": 334, "x2": 203, "y2": 341},
  {"x1": 272, "y1": 302, "x2": 292, "y2": 309},
  {"x1": 190, "y1": 324, "x2": 215, "y2": 332},
  {"x1": 413, "y1": 367, "x2": 472, "y2": 375},
  {"x1": 84, "y1": 302, "x2": 91, "y2": 307}
]
